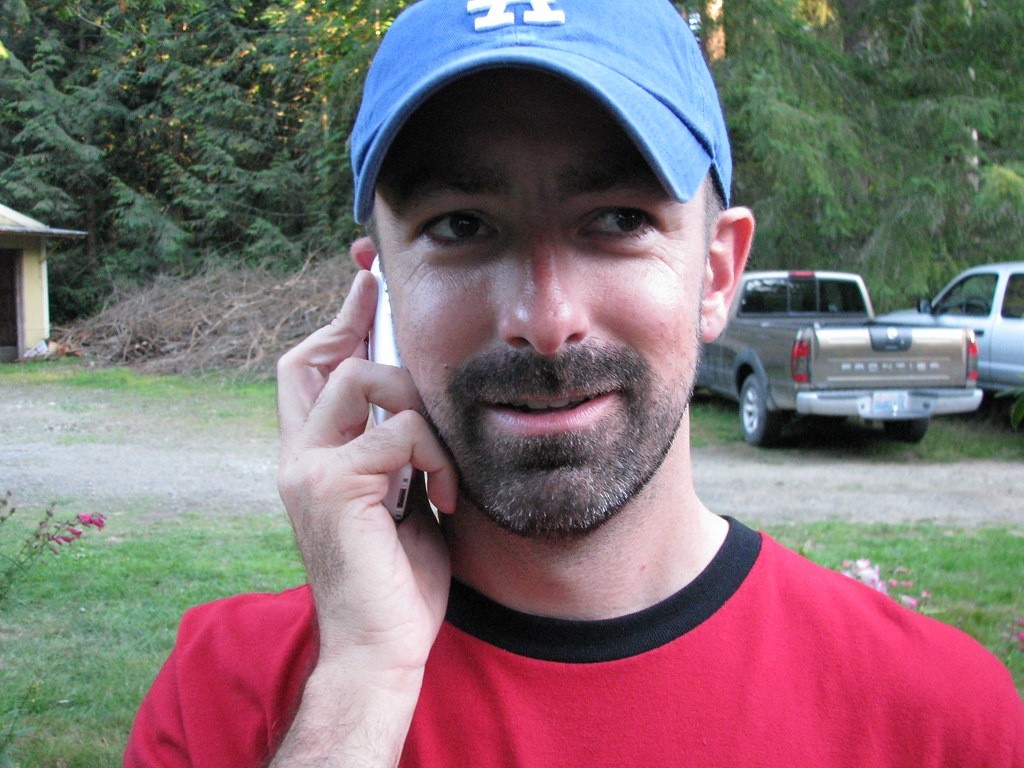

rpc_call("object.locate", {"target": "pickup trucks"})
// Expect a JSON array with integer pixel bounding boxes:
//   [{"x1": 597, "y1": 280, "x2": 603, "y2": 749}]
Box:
[{"x1": 692, "y1": 269, "x2": 985, "y2": 448}]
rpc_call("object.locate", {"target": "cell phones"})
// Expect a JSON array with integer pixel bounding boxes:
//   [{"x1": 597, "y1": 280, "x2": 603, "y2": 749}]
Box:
[{"x1": 352, "y1": 253, "x2": 412, "y2": 521}]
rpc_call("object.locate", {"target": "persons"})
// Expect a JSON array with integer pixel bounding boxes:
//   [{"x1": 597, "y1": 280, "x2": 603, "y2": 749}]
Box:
[{"x1": 124, "y1": 0, "x2": 1024, "y2": 768}]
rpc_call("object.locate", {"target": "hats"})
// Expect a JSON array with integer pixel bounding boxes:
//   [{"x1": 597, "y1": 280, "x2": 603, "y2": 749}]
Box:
[{"x1": 351, "y1": 0, "x2": 731, "y2": 224}]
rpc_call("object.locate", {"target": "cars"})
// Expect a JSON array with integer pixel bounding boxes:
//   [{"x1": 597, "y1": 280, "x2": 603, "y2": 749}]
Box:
[{"x1": 870, "y1": 259, "x2": 1024, "y2": 401}]
